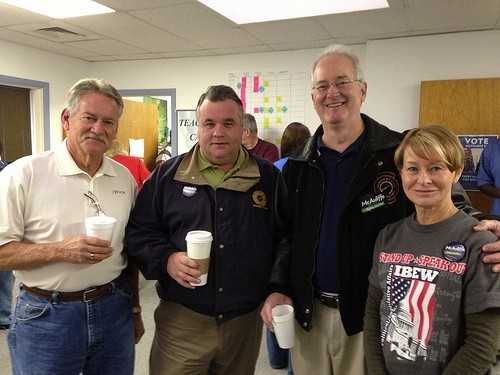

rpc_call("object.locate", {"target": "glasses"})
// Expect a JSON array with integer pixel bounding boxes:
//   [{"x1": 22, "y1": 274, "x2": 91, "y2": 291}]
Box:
[{"x1": 312, "y1": 79, "x2": 361, "y2": 92}]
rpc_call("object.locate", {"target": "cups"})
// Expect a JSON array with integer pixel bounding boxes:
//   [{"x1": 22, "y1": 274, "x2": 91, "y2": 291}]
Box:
[
  {"x1": 270, "y1": 304, "x2": 296, "y2": 349},
  {"x1": 83, "y1": 214, "x2": 116, "y2": 247},
  {"x1": 184, "y1": 230, "x2": 214, "y2": 287}
]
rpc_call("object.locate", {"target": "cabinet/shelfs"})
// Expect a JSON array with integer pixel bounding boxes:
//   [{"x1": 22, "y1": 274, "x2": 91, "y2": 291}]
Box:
[{"x1": 419, "y1": 78, "x2": 500, "y2": 214}]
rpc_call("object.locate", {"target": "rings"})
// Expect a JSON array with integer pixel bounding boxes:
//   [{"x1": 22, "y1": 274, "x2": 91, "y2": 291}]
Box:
[{"x1": 89, "y1": 253, "x2": 95, "y2": 259}]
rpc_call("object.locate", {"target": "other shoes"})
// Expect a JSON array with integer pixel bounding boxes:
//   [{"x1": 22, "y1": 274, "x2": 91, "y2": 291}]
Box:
[{"x1": 0, "y1": 325, "x2": 11, "y2": 330}]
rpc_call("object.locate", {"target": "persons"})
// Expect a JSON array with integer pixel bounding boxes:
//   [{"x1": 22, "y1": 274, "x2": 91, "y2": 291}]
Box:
[
  {"x1": 0, "y1": 146, "x2": 16, "y2": 330},
  {"x1": 266, "y1": 122, "x2": 310, "y2": 375},
  {"x1": 125, "y1": 85, "x2": 287, "y2": 375},
  {"x1": 475, "y1": 135, "x2": 500, "y2": 217},
  {"x1": 259, "y1": 43, "x2": 499, "y2": 375},
  {"x1": 0, "y1": 77, "x2": 146, "y2": 375},
  {"x1": 241, "y1": 113, "x2": 280, "y2": 163},
  {"x1": 104, "y1": 140, "x2": 151, "y2": 191},
  {"x1": 363, "y1": 125, "x2": 499, "y2": 375},
  {"x1": 155, "y1": 129, "x2": 172, "y2": 164}
]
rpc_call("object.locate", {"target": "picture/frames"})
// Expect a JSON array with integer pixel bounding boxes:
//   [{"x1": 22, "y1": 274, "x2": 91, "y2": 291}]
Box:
[{"x1": 176, "y1": 109, "x2": 199, "y2": 156}]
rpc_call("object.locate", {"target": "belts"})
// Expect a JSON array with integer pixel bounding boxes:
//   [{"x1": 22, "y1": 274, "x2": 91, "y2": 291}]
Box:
[
  {"x1": 314, "y1": 290, "x2": 340, "y2": 308},
  {"x1": 22, "y1": 271, "x2": 127, "y2": 303}
]
